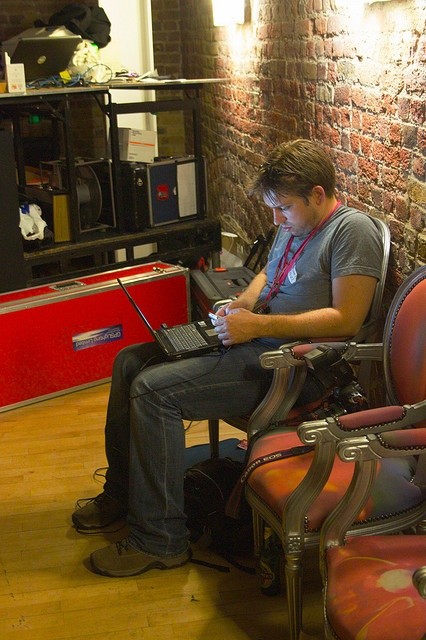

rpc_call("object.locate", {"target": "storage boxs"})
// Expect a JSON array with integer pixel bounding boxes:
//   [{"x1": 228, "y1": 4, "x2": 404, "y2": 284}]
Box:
[
  {"x1": 106, "y1": 128, "x2": 154, "y2": 164},
  {"x1": 0, "y1": 261, "x2": 191, "y2": 414}
]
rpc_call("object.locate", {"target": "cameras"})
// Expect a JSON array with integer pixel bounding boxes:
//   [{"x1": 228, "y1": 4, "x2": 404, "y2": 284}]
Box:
[{"x1": 303, "y1": 344, "x2": 371, "y2": 421}]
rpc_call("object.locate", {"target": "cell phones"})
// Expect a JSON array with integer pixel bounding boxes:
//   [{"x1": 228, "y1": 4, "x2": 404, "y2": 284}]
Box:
[{"x1": 208, "y1": 312, "x2": 222, "y2": 322}]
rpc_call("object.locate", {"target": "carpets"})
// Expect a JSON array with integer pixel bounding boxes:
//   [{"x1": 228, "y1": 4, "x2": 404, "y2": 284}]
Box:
[{"x1": 184, "y1": 438, "x2": 284, "y2": 567}]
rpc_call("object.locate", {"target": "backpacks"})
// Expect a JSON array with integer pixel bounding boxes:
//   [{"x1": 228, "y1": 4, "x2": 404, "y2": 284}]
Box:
[{"x1": 185, "y1": 458, "x2": 257, "y2": 575}]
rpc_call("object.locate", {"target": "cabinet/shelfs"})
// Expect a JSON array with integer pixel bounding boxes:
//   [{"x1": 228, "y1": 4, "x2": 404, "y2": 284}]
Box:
[{"x1": 1, "y1": 77, "x2": 231, "y2": 293}]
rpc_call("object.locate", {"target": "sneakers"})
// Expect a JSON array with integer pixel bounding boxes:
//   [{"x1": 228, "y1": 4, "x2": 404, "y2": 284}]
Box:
[
  {"x1": 73, "y1": 491, "x2": 128, "y2": 534},
  {"x1": 90, "y1": 534, "x2": 192, "y2": 577}
]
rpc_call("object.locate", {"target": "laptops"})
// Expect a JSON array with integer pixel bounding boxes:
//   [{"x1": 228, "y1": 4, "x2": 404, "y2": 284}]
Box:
[
  {"x1": 3, "y1": 35, "x2": 83, "y2": 86},
  {"x1": 116, "y1": 276, "x2": 224, "y2": 361}
]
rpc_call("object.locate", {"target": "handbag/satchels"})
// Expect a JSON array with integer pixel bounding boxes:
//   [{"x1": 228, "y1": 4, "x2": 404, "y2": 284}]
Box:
[{"x1": 48, "y1": 3, "x2": 111, "y2": 48}]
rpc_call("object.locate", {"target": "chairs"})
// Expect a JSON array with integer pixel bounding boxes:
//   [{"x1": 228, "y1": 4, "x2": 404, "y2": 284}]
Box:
[
  {"x1": 240, "y1": 267, "x2": 426, "y2": 640},
  {"x1": 317, "y1": 428, "x2": 426, "y2": 638},
  {"x1": 207, "y1": 221, "x2": 390, "y2": 460}
]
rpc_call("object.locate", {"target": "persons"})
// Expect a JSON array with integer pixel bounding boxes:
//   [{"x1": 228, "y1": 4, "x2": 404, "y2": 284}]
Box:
[{"x1": 73, "y1": 137, "x2": 383, "y2": 580}]
